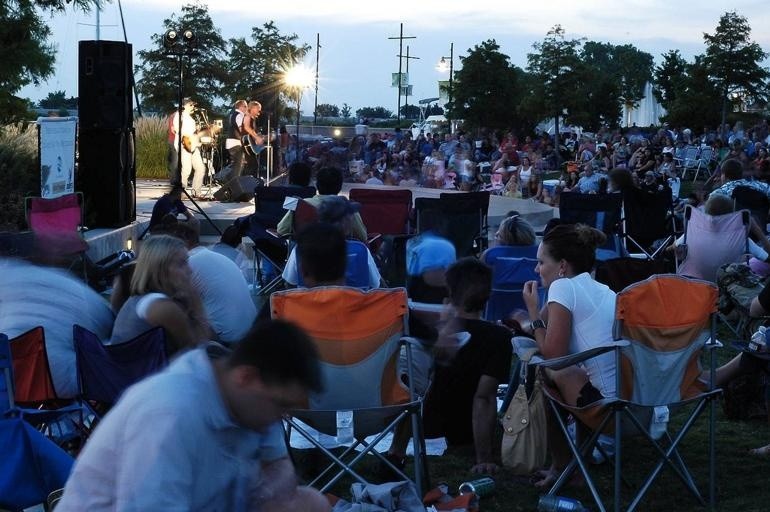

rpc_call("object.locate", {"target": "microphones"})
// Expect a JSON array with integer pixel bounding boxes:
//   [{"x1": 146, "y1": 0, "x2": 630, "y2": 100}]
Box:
[{"x1": 194, "y1": 107, "x2": 205, "y2": 111}]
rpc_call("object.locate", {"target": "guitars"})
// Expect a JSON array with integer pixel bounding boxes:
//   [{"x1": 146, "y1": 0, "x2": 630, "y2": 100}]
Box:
[
  {"x1": 183, "y1": 128, "x2": 219, "y2": 153},
  {"x1": 243, "y1": 130, "x2": 272, "y2": 159}
]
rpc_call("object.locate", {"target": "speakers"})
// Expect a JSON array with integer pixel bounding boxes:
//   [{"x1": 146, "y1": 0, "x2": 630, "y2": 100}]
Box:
[
  {"x1": 252, "y1": 81, "x2": 279, "y2": 129},
  {"x1": 78, "y1": 127, "x2": 136, "y2": 229},
  {"x1": 78, "y1": 40, "x2": 133, "y2": 129},
  {"x1": 259, "y1": 128, "x2": 278, "y2": 178},
  {"x1": 213, "y1": 175, "x2": 260, "y2": 203}
]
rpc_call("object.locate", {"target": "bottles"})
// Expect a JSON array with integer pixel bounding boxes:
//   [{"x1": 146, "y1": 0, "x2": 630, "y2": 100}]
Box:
[
  {"x1": 536, "y1": 493, "x2": 590, "y2": 512},
  {"x1": 747, "y1": 325, "x2": 769, "y2": 353}
]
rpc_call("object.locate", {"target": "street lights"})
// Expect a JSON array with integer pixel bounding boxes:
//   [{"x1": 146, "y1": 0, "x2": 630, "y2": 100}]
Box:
[{"x1": 435, "y1": 44, "x2": 455, "y2": 135}]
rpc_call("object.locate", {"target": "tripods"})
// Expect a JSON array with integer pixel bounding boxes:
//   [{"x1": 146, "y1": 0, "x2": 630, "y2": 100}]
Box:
[
  {"x1": 200, "y1": 111, "x2": 220, "y2": 200},
  {"x1": 137, "y1": 54, "x2": 223, "y2": 241}
]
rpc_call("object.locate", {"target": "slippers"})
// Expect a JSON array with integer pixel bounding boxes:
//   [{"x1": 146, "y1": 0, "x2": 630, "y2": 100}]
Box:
[
  {"x1": 748, "y1": 446, "x2": 770, "y2": 461},
  {"x1": 694, "y1": 377, "x2": 718, "y2": 393}
]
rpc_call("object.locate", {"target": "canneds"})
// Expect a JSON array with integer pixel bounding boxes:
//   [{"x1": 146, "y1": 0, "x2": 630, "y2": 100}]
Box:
[{"x1": 460, "y1": 478, "x2": 496, "y2": 502}]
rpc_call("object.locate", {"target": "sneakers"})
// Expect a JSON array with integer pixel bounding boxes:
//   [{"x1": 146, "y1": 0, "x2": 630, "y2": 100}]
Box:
[{"x1": 373, "y1": 451, "x2": 405, "y2": 474}]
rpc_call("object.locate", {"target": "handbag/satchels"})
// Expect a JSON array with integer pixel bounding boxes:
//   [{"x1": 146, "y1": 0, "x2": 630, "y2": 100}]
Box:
[{"x1": 500, "y1": 348, "x2": 548, "y2": 478}]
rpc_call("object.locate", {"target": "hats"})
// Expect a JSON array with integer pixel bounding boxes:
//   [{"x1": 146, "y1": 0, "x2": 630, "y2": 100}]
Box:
[
  {"x1": 319, "y1": 195, "x2": 360, "y2": 222},
  {"x1": 183, "y1": 97, "x2": 197, "y2": 106}
]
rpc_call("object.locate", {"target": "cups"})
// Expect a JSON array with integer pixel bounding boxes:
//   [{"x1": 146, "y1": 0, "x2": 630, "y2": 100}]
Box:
[{"x1": 458, "y1": 477, "x2": 498, "y2": 498}]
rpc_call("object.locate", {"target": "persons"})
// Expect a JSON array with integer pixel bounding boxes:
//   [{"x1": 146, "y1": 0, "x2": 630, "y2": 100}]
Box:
[
  {"x1": 50, "y1": 317, "x2": 332, "y2": 511},
  {"x1": 512, "y1": 222, "x2": 618, "y2": 489},
  {"x1": 367, "y1": 255, "x2": 516, "y2": 477},
  {"x1": 692, "y1": 278, "x2": 769, "y2": 459},
  {"x1": 0, "y1": 95, "x2": 769, "y2": 400}
]
